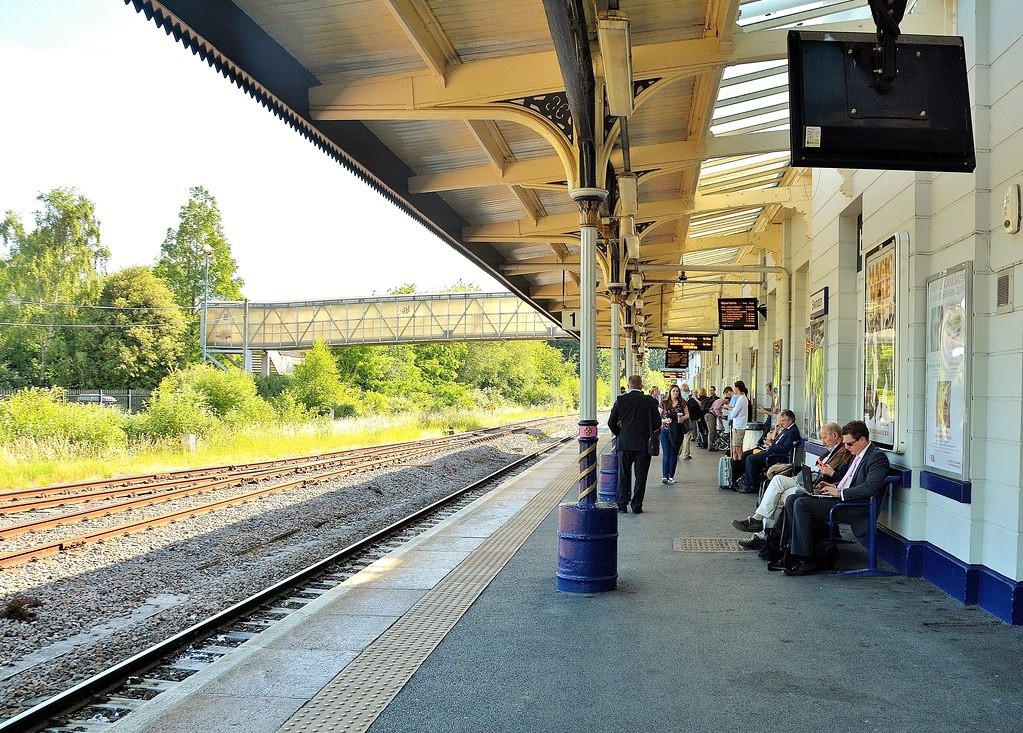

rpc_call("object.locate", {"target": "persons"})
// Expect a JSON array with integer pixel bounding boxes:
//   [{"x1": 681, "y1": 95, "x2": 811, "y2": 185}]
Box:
[
  {"x1": 608, "y1": 375, "x2": 662, "y2": 513},
  {"x1": 722, "y1": 380, "x2": 889, "y2": 576},
  {"x1": 620, "y1": 383, "x2": 730, "y2": 451},
  {"x1": 659, "y1": 384, "x2": 689, "y2": 484}
]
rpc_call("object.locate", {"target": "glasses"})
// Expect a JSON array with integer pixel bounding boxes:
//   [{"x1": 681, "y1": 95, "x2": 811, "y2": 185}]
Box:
[{"x1": 842, "y1": 437, "x2": 860, "y2": 446}]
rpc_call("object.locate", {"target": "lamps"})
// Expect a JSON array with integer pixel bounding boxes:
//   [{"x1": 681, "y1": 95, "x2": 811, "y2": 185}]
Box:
[
  {"x1": 620, "y1": 216, "x2": 635, "y2": 238},
  {"x1": 625, "y1": 292, "x2": 637, "y2": 306},
  {"x1": 636, "y1": 312, "x2": 647, "y2": 346},
  {"x1": 625, "y1": 232, "x2": 640, "y2": 259},
  {"x1": 635, "y1": 297, "x2": 644, "y2": 308},
  {"x1": 616, "y1": 171, "x2": 639, "y2": 214},
  {"x1": 630, "y1": 271, "x2": 643, "y2": 289},
  {"x1": 594, "y1": 8, "x2": 634, "y2": 117}
]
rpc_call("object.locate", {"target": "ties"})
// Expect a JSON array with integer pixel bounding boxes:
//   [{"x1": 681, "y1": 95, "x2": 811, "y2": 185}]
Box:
[
  {"x1": 837, "y1": 456, "x2": 859, "y2": 489},
  {"x1": 774, "y1": 429, "x2": 787, "y2": 442}
]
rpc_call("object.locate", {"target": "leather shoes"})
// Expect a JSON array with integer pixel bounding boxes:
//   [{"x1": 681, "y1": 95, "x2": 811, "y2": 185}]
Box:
[
  {"x1": 781, "y1": 561, "x2": 819, "y2": 575},
  {"x1": 767, "y1": 560, "x2": 792, "y2": 572}
]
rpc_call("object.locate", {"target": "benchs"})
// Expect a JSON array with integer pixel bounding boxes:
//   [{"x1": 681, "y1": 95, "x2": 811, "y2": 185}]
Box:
[
  {"x1": 765, "y1": 438, "x2": 800, "y2": 477},
  {"x1": 825, "y1": 474, "x2": 901, "y2": 578}
]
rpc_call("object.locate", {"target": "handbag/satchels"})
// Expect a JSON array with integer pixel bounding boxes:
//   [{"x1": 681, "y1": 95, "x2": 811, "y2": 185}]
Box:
[
  {"x1": 783, "y1": 538, "x2": 839, "y2": 571},
  {"x1": 686, "y1": 392, "x2": 702, "y2": 421},
  {"x1": 647, "y1": 433, "x2": 660, "y2": 456}
]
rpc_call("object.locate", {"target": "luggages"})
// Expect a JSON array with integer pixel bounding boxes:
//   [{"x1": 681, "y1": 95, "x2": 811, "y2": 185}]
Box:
[{"x1": 718, "y1": 455, "x2": 732, "y2": 489}]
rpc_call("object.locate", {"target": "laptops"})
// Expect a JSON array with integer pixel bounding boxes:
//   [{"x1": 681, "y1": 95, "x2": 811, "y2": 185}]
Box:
[{"x1": 800, "y1": 461, "x2": 837, "y2": 498}]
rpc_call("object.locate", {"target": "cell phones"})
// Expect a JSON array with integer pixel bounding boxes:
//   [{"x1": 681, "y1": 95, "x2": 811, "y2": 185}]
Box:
[
  {"x1": 817, "y1": 458, "x2": 826, "y2": 467},
  {"x1": 762, "y1": 437, "x2": 766, "y2": 441}
]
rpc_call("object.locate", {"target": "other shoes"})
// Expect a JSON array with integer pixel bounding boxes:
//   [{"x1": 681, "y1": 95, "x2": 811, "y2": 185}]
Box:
[
  {"x1": 732, "y1": 517, "x2": 764, "y2": 532},
  {"x1": 668, "y1": 478, "x2": 676, "y2": 483},
  {"x1": 690, "y1": 438, "x2": 719, "y2": 452},
  {"x1": 741, "y1": 486, "x2": 758, "y2": 493},
  {"x1": 633, "y1": 510, "x2": 643, "y2": 514},
  {"x1": 683, "y1": 456, "x2": 692, "y2": 460},
  {"x1": 737, "y1": 534, "x2": 764, "y2": 547},
  {"x1": 618, "y1": 508, "x2": 626, "y2": 513},
  {"x1": 759, "y1": 543, "x2": 782, "y2": 561},
  {"x1": 661, "y1": 477, "x2": 668, "y2": 483}
]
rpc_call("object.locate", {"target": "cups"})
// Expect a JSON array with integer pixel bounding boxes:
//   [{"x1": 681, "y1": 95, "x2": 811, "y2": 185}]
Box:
[{"x1": 677, "y1": 413, "x2": 683, "y2": 423}]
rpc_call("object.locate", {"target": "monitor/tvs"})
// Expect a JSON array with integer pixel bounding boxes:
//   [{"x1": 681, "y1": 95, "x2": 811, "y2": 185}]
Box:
[
  {"x1": 786, "y1": 29, "x2": 977, "y2": 175},
  {"x1": 665, "y1": 351, "x2": 688, "y2": 368},
  {"x1": 718, "y1": 297, "x2": 761, "y2": 331}
]
